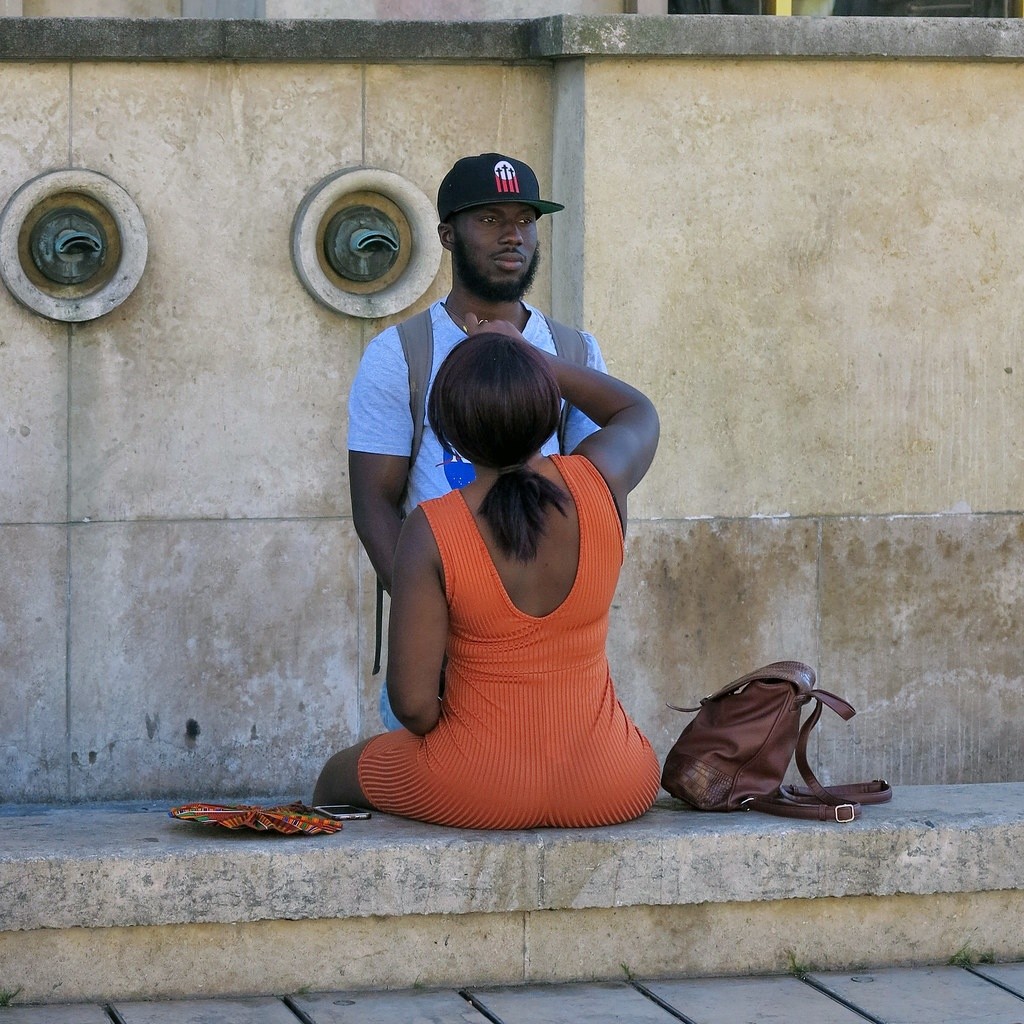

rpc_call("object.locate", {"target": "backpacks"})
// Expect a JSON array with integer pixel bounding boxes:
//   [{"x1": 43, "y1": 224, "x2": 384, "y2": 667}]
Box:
[{"x1": 660, "y1": 660, "x2": 892, "y2": 823}]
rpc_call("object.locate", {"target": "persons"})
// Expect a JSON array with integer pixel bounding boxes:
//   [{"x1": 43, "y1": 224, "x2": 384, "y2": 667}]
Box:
[
  {"x1": 314, "y1": 312, "x2": 661, "y2": 829},
  {"x1": 347, "y1": 152, "x2": 611, "y2": 739}
]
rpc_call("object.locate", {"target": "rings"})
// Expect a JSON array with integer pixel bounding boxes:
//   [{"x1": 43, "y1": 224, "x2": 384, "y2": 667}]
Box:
[{"x1": 478, "y1": 319, "x2": 488, "y2": 326}]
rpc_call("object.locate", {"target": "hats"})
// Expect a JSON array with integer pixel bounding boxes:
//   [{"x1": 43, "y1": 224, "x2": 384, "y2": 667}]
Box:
[{"x1": 437, "y1": 153, "x2": 565, "y2": 221}]
